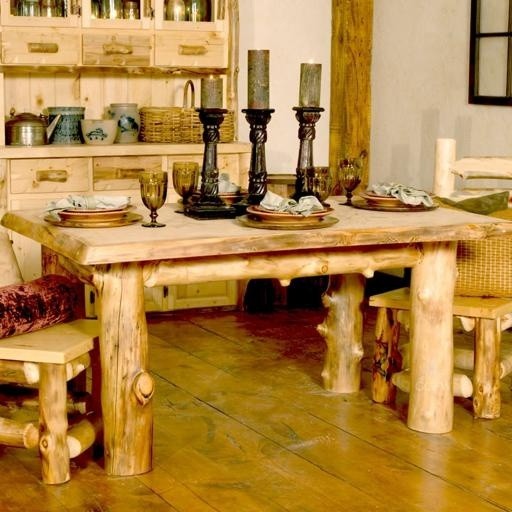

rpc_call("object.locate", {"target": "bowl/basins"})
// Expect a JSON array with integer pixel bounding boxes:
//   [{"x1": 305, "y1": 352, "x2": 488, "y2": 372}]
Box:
[{"x1": 81, "y1": 119, "x2": 118, "y2": 146}]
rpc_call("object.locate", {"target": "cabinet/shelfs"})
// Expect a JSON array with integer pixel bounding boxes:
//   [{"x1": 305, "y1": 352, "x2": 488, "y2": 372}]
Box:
[{"x1": 0, "y1": 0, "x2": 253, "y2": 318}]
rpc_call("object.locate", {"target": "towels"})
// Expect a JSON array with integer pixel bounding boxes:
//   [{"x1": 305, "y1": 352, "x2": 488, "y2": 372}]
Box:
[
  {"x1": 39, "y1": 194, "x2": 130, "y2": 222},
  {"x1": 260, "y1": 191, "x2": 324, "y2": 217},
  {"x1": 368, "y1": 181, "x2": 433, "y2": 208},
  {"x1": 219, "y1": 174, "x2": 241, "y2": 192}
]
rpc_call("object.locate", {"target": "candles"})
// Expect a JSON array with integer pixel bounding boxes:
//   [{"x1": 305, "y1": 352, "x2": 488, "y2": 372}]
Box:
[
  {"x1": 200, "y1": 76, "x2": 223, "y2": 107},
  {"x1": 300, "y1": 62, "x2": 322, "y2": 108},
  {"x1": 248, "y1": 50, "x2": 270, "y2": 108}
]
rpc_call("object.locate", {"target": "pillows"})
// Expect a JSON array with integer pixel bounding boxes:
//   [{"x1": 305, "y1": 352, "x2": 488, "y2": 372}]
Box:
[
  {"x1": 454, "y1": 208, "x2": 512, "y2": 299},
  {"x1": 427, "y1": 190, "x2": 509, "y2": 215}
]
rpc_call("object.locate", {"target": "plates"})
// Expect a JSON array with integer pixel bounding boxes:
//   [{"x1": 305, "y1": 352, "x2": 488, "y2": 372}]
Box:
[
  {"x1": 361, "y1": 193, "x2": 438, "y2": 206},
  {"x1": 44, "y1": 215, "x2": 144, "y2": 228},
  {"x1": 239, "y1": 215, "x2": 339, "y2": 230},
  {"x1": 246, "y1": 205, "x2": 334, "y2": 222},
  {"x1": 352, "y1": 200, "x2": 440, "y2": 212},
  {"x1": 57, "y1": 205, "x2": 135, "y2": 223}
]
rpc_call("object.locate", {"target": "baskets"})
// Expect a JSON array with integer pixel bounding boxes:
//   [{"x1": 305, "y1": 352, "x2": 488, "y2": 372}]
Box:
[
  {"x1": 139, "y1": 80, "x2": 234, "y2": 144},
  {"x1": 442, "y1": 207, "x2": 511, "y2": 299}
]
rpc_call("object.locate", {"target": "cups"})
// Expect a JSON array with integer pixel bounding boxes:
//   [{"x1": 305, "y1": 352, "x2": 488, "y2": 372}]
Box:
[
  {"x1": 337, "y1": 157, "x2": 363, "y2": 206},
  {"x1": 305, "y1": 167, "x2": 331, "y2": 207},
  {"x1": 173, "y1": 162, "x2": 199, "y2": 214},
  {"x1": 140, "y1": 172, "x2": 169, "y2": 228}
]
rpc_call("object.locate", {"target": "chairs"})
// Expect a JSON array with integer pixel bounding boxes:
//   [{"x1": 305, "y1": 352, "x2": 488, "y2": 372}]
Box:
[
  {"x1": 368, "y1": 137, "x2": 512, "y2": 420},
  {"x1": 0, "y1": 229, "x2": 100, "y2": 487}
]
